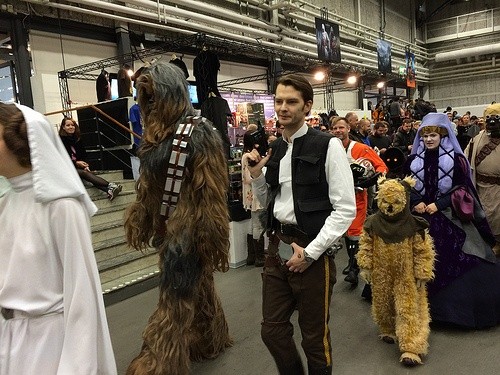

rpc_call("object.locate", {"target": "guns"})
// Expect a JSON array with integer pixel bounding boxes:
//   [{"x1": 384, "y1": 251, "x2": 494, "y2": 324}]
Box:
[{"x1": 247, "y1": 120, "x2": 268, "y2": 167}]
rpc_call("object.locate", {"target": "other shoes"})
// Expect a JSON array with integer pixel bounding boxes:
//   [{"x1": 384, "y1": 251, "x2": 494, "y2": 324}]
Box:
[
  {"x1": 342, "y1": 259, "x2": 360, "y2": 285},
  {"x1": 399, "y1": 351, "x2": 423, "y2": 367},
  {"x1": 383, "y1": 334, "x2": 397, "y2": 344}
]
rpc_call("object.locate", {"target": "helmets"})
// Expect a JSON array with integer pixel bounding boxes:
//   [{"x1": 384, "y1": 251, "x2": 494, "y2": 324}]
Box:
[{"x1": 350, "y1": 157, "x2": 375, "y2": 191}]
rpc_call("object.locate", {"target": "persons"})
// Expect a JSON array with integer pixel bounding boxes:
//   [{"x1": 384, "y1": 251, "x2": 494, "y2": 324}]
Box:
[
  {"x1": 318, "y1": 23, "x2": 339, "y2": 61},
  {"x1": 57, "y1": 115, "x2": 123, "y2": 201},
  {"x1": 328, "y1": 114, "x2": 388, "y2": 289},
  {"x1": 234, "y1": 98, "x2": 500, "y2": 268},
  {"x1": 407, "y1": 112, "x2": 499, "y2": 328},
  {"x1": 242, "y1": 73, "x2": 356, "y2": 374},
  {"x1": 0, "y1": 101, "x2": 119, "y2": 375}
]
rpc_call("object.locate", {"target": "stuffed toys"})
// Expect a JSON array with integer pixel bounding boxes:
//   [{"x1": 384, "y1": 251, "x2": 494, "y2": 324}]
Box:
[
  {"x1": 355, "y1": 176, "x2": 440, "y2": 364},
  {"x1": 123, "y1": 59, "x2": 234, "y2": 375}
]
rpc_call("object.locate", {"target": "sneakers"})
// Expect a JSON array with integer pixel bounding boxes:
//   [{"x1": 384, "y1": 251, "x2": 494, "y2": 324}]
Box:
[{"x1": 107, "y1": 183, "x2": 122, "y2": 201}]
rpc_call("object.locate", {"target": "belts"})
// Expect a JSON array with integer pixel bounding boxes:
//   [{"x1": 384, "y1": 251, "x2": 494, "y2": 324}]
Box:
[{"x1": 271, "y1": 214, "x2": 309, "y2": 241}]
